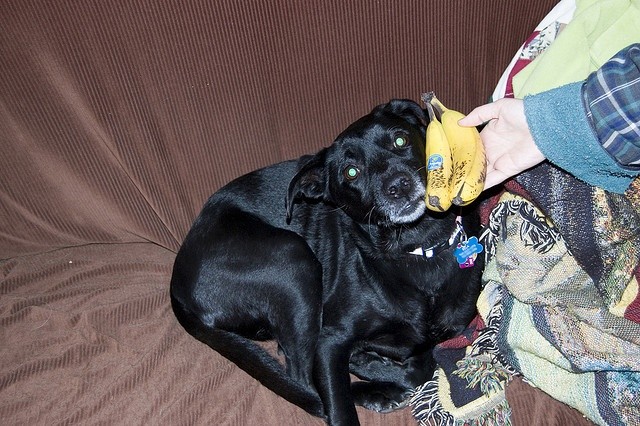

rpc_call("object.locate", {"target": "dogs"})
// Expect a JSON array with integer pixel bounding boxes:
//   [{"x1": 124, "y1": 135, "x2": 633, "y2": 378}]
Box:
[{"x1": 168, "y1": 96, "x2": 487, "y2": 426}]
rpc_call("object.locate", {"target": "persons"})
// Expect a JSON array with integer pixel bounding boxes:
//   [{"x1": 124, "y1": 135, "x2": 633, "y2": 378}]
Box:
[{"x1": 457, "y1": 43, "x2": 639, "y2": 194}]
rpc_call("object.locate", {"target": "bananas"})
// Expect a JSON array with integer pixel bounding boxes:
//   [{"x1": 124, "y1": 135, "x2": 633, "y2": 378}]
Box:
[{"x1": 424, "y1": 90, "x2": 488, "y2": 213}]
